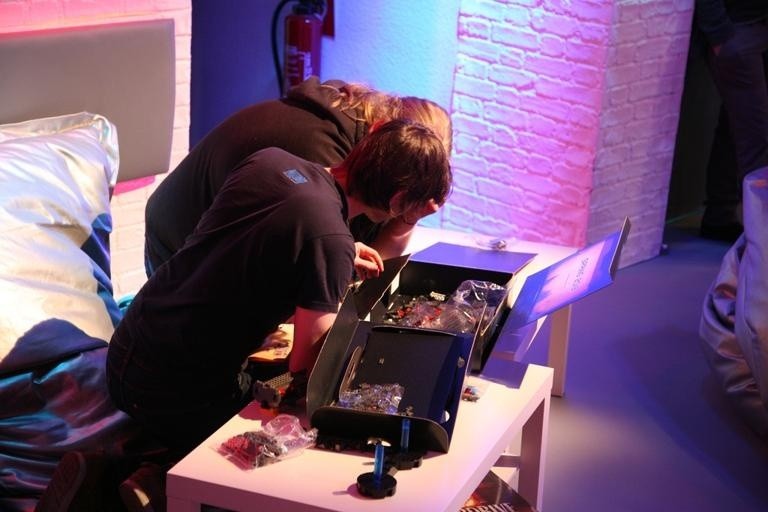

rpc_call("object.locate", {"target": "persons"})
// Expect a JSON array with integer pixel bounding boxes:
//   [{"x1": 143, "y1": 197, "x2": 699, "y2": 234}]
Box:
[
  {"x1": 35, "y1": 117, "x2": 452, "y2": 512},
  {"x1": 143, "y1": 78, "x2": 453, "y2": 350}
]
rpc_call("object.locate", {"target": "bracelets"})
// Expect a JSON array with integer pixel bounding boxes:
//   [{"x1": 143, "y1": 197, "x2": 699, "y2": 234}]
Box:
[{"x1": 401, "y1": 217, "x2": 415, "y2": 226}]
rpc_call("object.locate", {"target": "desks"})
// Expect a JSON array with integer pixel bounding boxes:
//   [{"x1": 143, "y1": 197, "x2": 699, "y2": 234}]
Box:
[
  {"x1": 388, "y1": 225, "x2": 580, "y2": 397},
  {"x1": 166, "y1": 364, "x2": 553, "y2": 510}
]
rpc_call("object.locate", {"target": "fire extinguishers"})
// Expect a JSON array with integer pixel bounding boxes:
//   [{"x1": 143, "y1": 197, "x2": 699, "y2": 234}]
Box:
[{"x1": 269, "y1": 1, "x2": 322, "y2": 99}]
